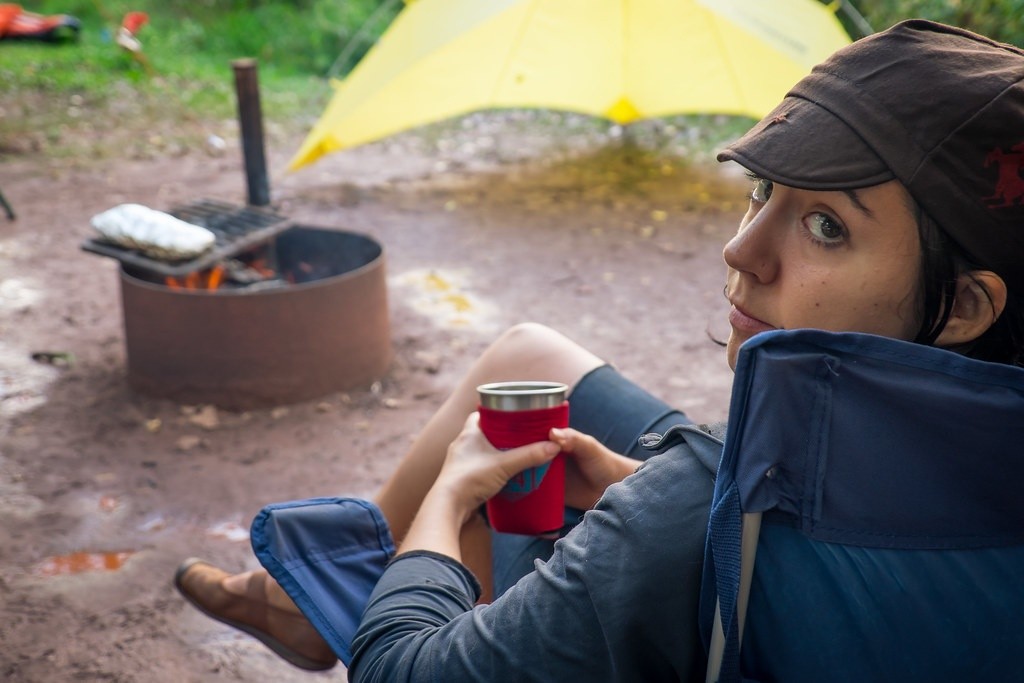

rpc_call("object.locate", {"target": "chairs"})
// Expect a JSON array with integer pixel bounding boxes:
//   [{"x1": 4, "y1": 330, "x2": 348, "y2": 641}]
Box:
[{"x1": 692, "y1": 327, "x2": 1024, "y2": 683}]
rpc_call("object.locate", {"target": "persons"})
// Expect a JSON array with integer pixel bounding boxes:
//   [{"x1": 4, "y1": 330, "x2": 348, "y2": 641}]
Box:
[{"x1": 173, "y1": 18, "x2": 1024, "y2": 682}]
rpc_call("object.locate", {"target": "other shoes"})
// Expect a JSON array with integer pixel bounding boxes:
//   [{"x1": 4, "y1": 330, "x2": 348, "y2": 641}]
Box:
[{"x1": 172, "y1": 557, "x2": 337, "y2": 670}]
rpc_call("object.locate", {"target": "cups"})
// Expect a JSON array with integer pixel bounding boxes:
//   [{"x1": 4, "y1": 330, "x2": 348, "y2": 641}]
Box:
[{"x1": 477, "y1": 381, "x2": 569, "y2": 536}]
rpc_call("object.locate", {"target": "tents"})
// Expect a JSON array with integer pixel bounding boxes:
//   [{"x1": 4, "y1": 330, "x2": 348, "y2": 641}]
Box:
[{"x1": 263, "y1": 0, "x2": 852, "y2": 186}]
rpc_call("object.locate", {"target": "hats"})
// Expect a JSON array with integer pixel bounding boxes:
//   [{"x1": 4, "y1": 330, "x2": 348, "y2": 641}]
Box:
[{"x1": 716, "y1": 19, "x2": 1024, "y2": 287}]
